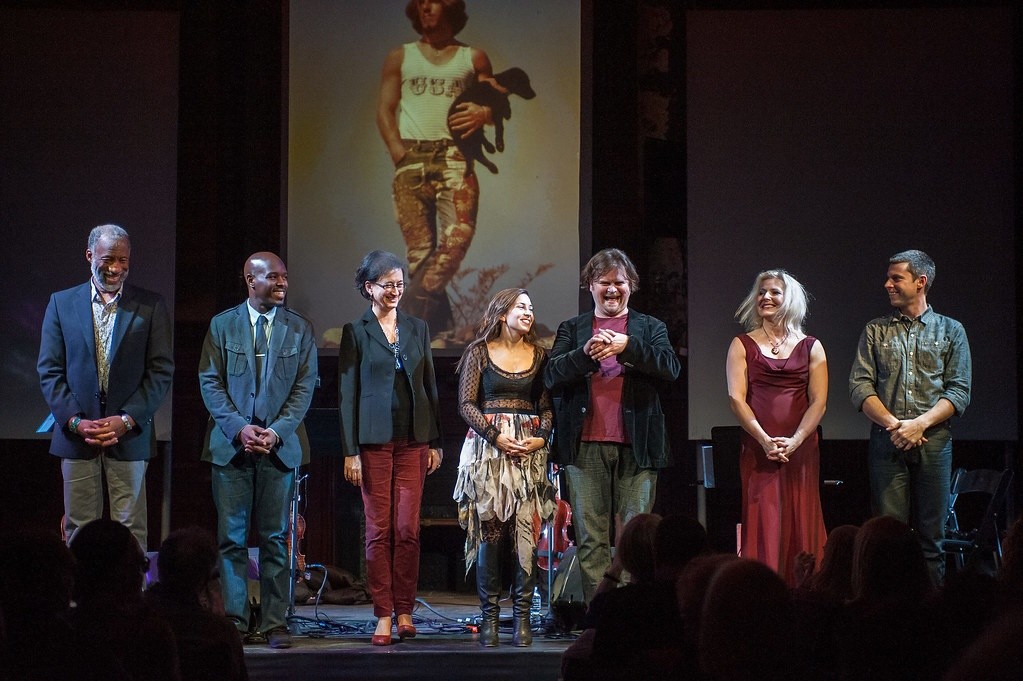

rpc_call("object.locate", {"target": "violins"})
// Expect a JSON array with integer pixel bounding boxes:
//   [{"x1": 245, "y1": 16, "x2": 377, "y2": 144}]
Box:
[
  {"x1": 536, "y1": 464, "x2": 572, "y2": 572},
  {"x1": 288, "y1": 500, "x2": 306, "y2": 582}
]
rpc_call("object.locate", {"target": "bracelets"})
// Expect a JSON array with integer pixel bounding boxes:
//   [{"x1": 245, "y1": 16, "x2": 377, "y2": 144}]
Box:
[
  {"x1": 482, "y1": 105, "x2": 487, "y2": 119},
  {"x1": 121, "y1": 415, "x2": 132, "y2": 430},
  {"x1": 604, "y1": 574, "x2": 620, "y2": 583},
  {"x1": 70, "y1": 417, "x2": 81, "y2": 433}
]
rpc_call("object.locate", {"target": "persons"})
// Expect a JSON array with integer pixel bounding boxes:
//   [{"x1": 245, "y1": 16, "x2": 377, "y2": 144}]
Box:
[
  {"x1": 544, "y1": 249, "x2": 682, "y2": 606},
  {"x1": 848, "y1": 250, "x2": 972, "y2": 599},
  {"x1": 37, "y1": 225, "x2": 175, "y2": 555},
  {"x1": 561, "y1": 513, "x2": 1023, "y2": 681},
  {"x1": 452, "y1": 288, "x2": 558, "y2": 647},
  {"x1": 198, "y1": 252, "x2": 318, "y2": 647},
  {"x1": 726, "y1": 270, "x2": 828, "y2": 589},
  {"x1": 0, "y1": 517, "x2": 249, "y2": 681},
  {"x1": 376, "y1": 0, "x2": 492, "y2": 339},
  {"x1": 337, "y1": 249, "x2": 444, "y2": 645}
]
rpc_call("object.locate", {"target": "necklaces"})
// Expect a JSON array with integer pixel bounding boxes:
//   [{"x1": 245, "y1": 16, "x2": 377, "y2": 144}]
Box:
[
  {"x1": 433, "y1": 48, "x2": 440, "y2": 57},
  {"x1": 763, "y1": 326, "x2": 788, "y2": 355}
]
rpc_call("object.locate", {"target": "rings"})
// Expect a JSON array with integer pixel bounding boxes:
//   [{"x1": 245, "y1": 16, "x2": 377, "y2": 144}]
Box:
[
  {"x1": 901, "y1": 441, "x2": 905, "y2": 445},
  {"x1": 437, "y1": 465, "x2": 440, "y2": 468}
]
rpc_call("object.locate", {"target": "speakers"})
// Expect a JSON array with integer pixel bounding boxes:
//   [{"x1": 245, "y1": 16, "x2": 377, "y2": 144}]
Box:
[{"x1": 550, "y1": 545, "x2": 587, "y2": 604}]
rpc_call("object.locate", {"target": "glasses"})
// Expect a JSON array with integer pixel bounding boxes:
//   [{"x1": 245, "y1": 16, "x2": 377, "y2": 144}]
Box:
[{"x1": 372, "y1": 283, "x2": 407, "y2": 292}]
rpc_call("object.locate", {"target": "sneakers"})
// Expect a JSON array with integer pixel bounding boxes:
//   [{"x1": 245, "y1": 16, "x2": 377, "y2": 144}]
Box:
[
  {"x1": 239, "y1": 632, "x2": 249, "y2": 645},
  {"x1": 266, "y1": 627, "x2": 291, "y2": 648}
]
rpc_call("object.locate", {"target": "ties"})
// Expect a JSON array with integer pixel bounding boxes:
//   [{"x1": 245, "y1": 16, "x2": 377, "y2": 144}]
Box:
[{"x1": 253, "y1": 316, "x2": 268, "y2": 384}]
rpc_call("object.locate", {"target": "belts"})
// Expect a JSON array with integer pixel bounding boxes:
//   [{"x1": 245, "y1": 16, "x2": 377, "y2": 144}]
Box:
[{"x1": 410, "y1": 140, "x2": 456, "y2": 148}]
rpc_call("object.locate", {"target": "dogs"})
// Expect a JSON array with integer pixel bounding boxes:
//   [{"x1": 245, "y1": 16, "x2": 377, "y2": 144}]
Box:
[{"x1": 447, "y1": 66, "x2": 537, "y2": 178}]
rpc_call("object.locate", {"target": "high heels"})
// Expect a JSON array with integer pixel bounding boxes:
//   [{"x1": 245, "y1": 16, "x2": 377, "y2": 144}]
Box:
[
  {"x1": 395, "y1": 620, "x2": 417, "y2": 643},
  {"x1": 372, "y1": 623, "x2": 393, "y2": 645}
]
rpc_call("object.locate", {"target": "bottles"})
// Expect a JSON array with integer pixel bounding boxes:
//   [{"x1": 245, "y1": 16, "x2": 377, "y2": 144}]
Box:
[{"x1": 529, "y1": 586, "x2": 541, "y2": 632}]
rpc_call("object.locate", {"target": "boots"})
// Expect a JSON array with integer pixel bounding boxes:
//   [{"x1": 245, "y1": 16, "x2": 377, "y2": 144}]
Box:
[
  {"x1": 513, "y1": 554, "x2": 532, "y2": 647},
  {"x1": 477, "y1": 542, "x2": 501, "y2": 646}
]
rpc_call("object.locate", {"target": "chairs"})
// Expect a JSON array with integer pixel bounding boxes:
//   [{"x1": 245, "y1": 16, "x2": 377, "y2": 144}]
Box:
[
  {"x1": 942, "y1": 467, "x2": 1014, "y2": 570},
  {"x1": 180, "y1": 637, "x2": 240, "y2": 681},
  {"x1": 711, "y1": 424, "x2": 826, "y2": 557}
]
